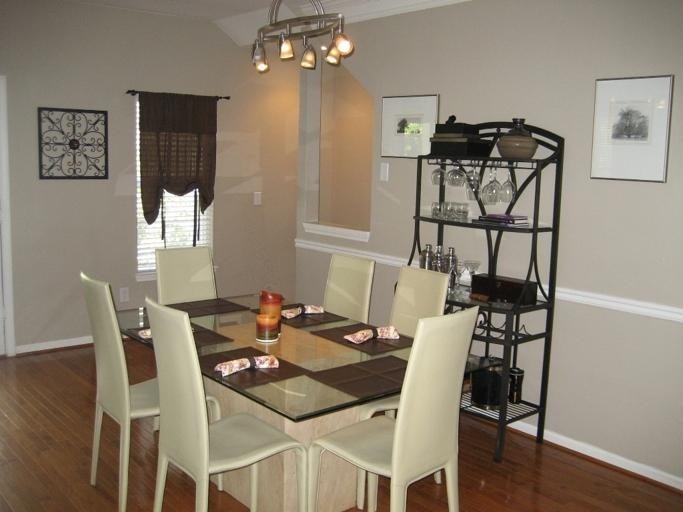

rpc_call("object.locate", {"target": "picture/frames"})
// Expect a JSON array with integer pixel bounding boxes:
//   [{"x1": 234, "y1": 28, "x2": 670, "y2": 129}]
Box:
[
  {"x1": 590, "y1": 74, "x2": 675, "y2": 184},
  {"x1": 380, "y1": 93, "x2": 440, "y2": 161},
  {"x1": 36, "y1": 107, "x2": 108, "y2": 180}
]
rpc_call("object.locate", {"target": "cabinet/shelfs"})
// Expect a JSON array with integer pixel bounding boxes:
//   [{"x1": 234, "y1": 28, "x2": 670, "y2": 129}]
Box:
[{"x1": 389, "y1": 119, "x2": 567, "y2": 463}]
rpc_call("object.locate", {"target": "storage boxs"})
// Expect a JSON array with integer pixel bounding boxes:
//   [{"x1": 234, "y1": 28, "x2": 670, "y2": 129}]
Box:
[
  {"x1": 431, "y1": 122, "x2": 478, "y2": 138},
  {"x1": 469, "y1": 272, "x2": 539, "y2": 307},
  {"x1": 428, "y1": 137, "x2": 491, "y2": 159}
]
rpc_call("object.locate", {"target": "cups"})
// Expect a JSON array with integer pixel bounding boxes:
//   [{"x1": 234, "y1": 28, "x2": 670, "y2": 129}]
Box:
[
  {"x1": 258, "y1": 289, "x2": 283, "y2": 335},
  {"x1": 255, "y1": 314, "x2": 279, "y2": 343},
  {"x1": 508, "y1": 367, "x2": 523, "y2": 403},
  {"x1": 430, "y1": 201, "x2": 468, "y2": 219}
]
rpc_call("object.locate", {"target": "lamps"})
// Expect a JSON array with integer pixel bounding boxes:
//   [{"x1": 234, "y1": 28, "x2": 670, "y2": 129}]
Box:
[{"x1": 250, "y1": 1, "x2": 354, "y2": 74}]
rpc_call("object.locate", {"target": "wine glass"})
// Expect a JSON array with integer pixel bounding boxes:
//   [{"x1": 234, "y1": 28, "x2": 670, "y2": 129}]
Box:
[{"x1": 429, "y1": 162, "x2": 516, "y2": 205}]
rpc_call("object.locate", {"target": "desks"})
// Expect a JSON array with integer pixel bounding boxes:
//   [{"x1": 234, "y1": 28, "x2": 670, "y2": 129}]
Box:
[{"x1": 105, "y1": 293, "x2": 421, "y2": 512}]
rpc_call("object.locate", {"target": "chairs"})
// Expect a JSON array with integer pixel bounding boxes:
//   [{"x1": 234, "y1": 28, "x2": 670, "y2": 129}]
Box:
[
  {"x1": 304, "y1": 303, "x2": 482, "y2": 511},
  {"x1": 75, "y1": 269, "x2": 225, "y2": 496},
  {"x1": 319, "y1": 249, "x2": 374, "y2": 328},
  {"x1": 139, "y1": 296, "x2": 309, "y2": 511},
  {"x1": 151, "y1": 243, "x2": 218, "y2": 305},
  {"x1": 383, "y1": 264, "x2": 452, "y2": 485}
]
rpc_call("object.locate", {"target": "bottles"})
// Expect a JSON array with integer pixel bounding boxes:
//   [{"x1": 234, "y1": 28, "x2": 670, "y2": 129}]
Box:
[
  {"x1": 468, "y1": 356, "x2": 506, "y2": 409},
  {"x1": 419, "y1": 243, "x2": 455, "y2": 292},
  {"x1": 497, "y1": 117, "x2": 537, "y2": 161}
]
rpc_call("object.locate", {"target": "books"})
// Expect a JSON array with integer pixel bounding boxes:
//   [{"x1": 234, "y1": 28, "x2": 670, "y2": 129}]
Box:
[{"x1": 471, "y1": 214, "x2": 529, "y2": 228}]
